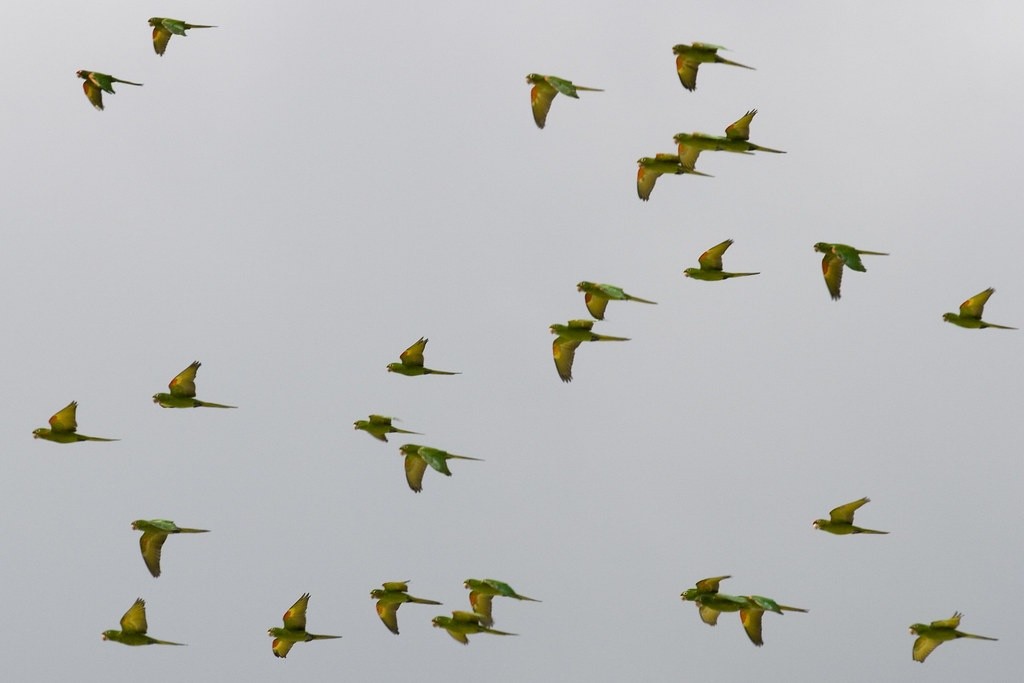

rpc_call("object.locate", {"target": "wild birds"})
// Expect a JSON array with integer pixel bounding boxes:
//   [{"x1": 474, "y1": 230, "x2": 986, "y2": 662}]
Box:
[
  {"x1": 908, "y1": 609, "x2": 1001, "y2": 663},
  {"x1": 811, "y1": 498, "x2": 891, "y2": 538},
  {"x1": 369, "y1": 581, "x2": 445, "y2": 636},
  {"x1": 679, "y1": 574, "x2": 811, "y2": 648},
  {"x1": 147, "y1": 16, "x2": 219, "y2": 57},
  {"x1": 353, "y1": 412, "x2": 426, "y2": 442},
  {"x1": 634, "y1": 109, "x2": 790, "y2": 202},
  {"x1": 31, "y1": 398, "x2": 118, "y2": 443},
  {"x1": 942, "y1": 288, "x2": 1020, "y2": 333},
  {"x1": 265, "y1": 592, "x2": 344, "y2": 660},
  {"x1": 386, "y1": 337, "x2": 466, "y2": 379},
  {"x1": 813, "y1": 240, "x2": 891, "y2": 302},
  {"x1": 399, "y1": 443, "x2": 486, "y2": 493},
  {"x1": 131, "y1": 517, "x2": 211, "y2": 578},
  {"x1": 149, "y1": 360, "x2": 240, "y2": 409},
  {"x1": 670, "y1": 41, "x2": 757, "y2": 92},
  {"x1": 100, "y1": 599, "x2": 183, "y2": 649},
  {"x1": 430, "y1": 573, "x2": 544, "y2": 645},
  {"x1": 526, "y1": 73, "x2": 605, "y2": 130},
  {"x1": 548, "y1": 280, "x2": 658, "y2": 385},
  {"x1": 76, "y1": 70, "x2": 144, "y2": 112},
  {"x1": 682, "y1": 238, "x2": 761, "y2": 281}
]
